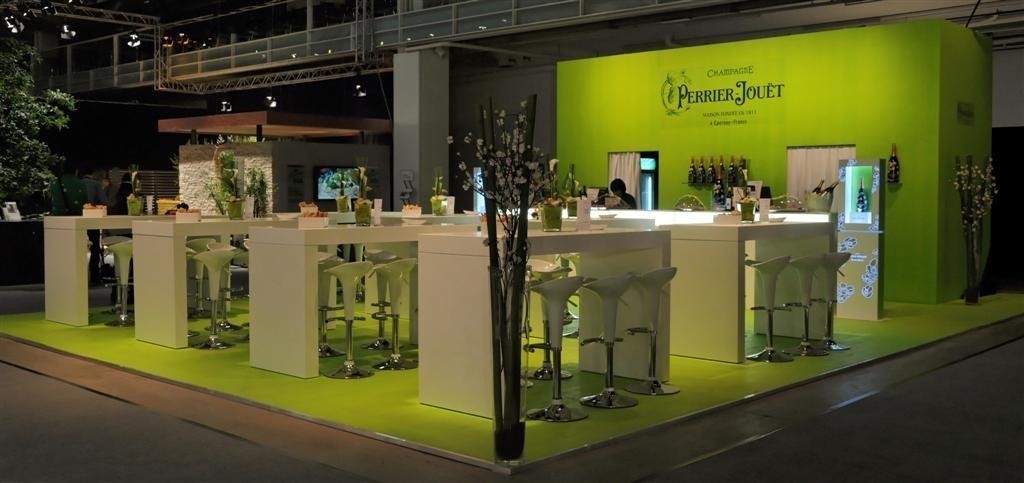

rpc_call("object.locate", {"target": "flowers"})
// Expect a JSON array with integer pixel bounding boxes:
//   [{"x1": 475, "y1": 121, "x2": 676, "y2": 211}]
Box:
[
  {"x1": 431, "y1": 175, "x2": 446, "y2": 198},
  {"x1": 543, "y1": 157, "x2": 562, "y2": 206},
  {"x1": 226, "y1": 168, "x2": 241, "y2": 202},
  {"x1": 949, "y1": 154, "x2": 998, "y2": 285},
  {"x1": 737, "y1": 167, "x2": 758, "y2": 203},
  {"x1": 447, "y1": 91, "x2": 551, "y2": 424},
  {"x1": 356, "y1": 167, "x2": 372, "y2": 203},
  {"x1": 127, "y1": 170, "x2": 144, "y2": 199},
  {"x1": 566, "y1": 165, "x2": 581, "y2": 201},
  {"x1": 336, "y1": 169, "x2": 351, "y2": 198}
]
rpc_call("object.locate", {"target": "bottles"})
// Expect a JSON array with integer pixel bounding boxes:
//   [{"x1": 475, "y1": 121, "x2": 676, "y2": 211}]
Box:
[
  {"x1": 887, "y1": 142, "x2": 900, "y2": 183},
  {"x1": 819, "y1": 181, "x2": 839, "y2": 198},
  {"x1": 856, "y1": 176, "x2": 867, "y2": 212},
  {"x1": 727, "y1": 154, "x2": 736, "y2": 186},
  {"x1": 688, "y1": 155, "x2": 696, "y2": 184},
  {"x1": 812, "y1": 179, "x2": 824, "y2": 195},
  {"x1": 706, "y1": 154, "x2": 715, "y2": 184},
  {"x1": 736, "y1": 153, "x2": 748, "y2": 187},
  {"x1": 725, "y1": 186, "x2": 735, "y2": 211},
  {"x1": 696, "y1": 154, "x2": 705, "y2": 184},
  {"x1": 716, "y1": 154, "x2": 726, "y2": 183}
]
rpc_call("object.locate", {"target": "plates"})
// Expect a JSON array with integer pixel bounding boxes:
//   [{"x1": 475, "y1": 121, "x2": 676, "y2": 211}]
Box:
[
  {"x1": 591, "y1": 225, "x2": 609, "y2": 230},
  {"x1": 401, "y1": 219, "x2": 427, "y2": 225},
  {"x1": 598, "y1": 214, "x2": 618, "y2": 219}
]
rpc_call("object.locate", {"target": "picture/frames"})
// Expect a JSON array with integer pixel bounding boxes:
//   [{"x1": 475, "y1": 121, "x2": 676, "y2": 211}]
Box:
[{"x1": 747, "y1": 180, "x2": 763, "y2": 200}]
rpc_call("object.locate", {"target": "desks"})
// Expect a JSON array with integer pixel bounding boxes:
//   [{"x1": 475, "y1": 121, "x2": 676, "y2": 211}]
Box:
[{"x1": 1, "y1": 220, "x2": 102, "y2": 287}]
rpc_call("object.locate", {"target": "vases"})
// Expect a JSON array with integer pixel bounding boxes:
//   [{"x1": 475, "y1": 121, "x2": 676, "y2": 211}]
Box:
[
  {"x1": 335, "y1": 197, "x2": 351, "y2": 211},
  {"x1": 431, "y1": 198, "x2": 448, "y2": 215},
  {"x1": 961, "y1": 220, "x2": 984, "y2": 305},
  {"x1": 487, "y1": 269, "x2": 529, "y2": 466}
]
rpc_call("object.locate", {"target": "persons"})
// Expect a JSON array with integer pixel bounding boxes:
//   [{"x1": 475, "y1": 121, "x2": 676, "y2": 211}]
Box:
[
  {"x1": 101, "y1": 177, "x2": 109, "y2": 191},
  {"x1": 114, "y1": 172, "x2": 137, "y2": 217},
  {"x1": 76, "y1": 172, "x2": 107, "y2": 288},
  {"x1": 609, "y1": 177, "x2": 636, "y2": 209}
]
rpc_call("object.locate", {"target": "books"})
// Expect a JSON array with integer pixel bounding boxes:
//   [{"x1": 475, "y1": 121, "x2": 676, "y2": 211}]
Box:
[{"x1": 0, "y1": 201, "x2": 22, "y2": 222}]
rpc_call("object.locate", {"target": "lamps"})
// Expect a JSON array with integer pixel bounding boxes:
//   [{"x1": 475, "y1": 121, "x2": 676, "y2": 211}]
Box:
[
  {"x1": 4, "y1": 0, "x2": 211, "y2": 55},
  {"x1": 220, "y1": 76, "x2": 367, "y2": 114}
]
